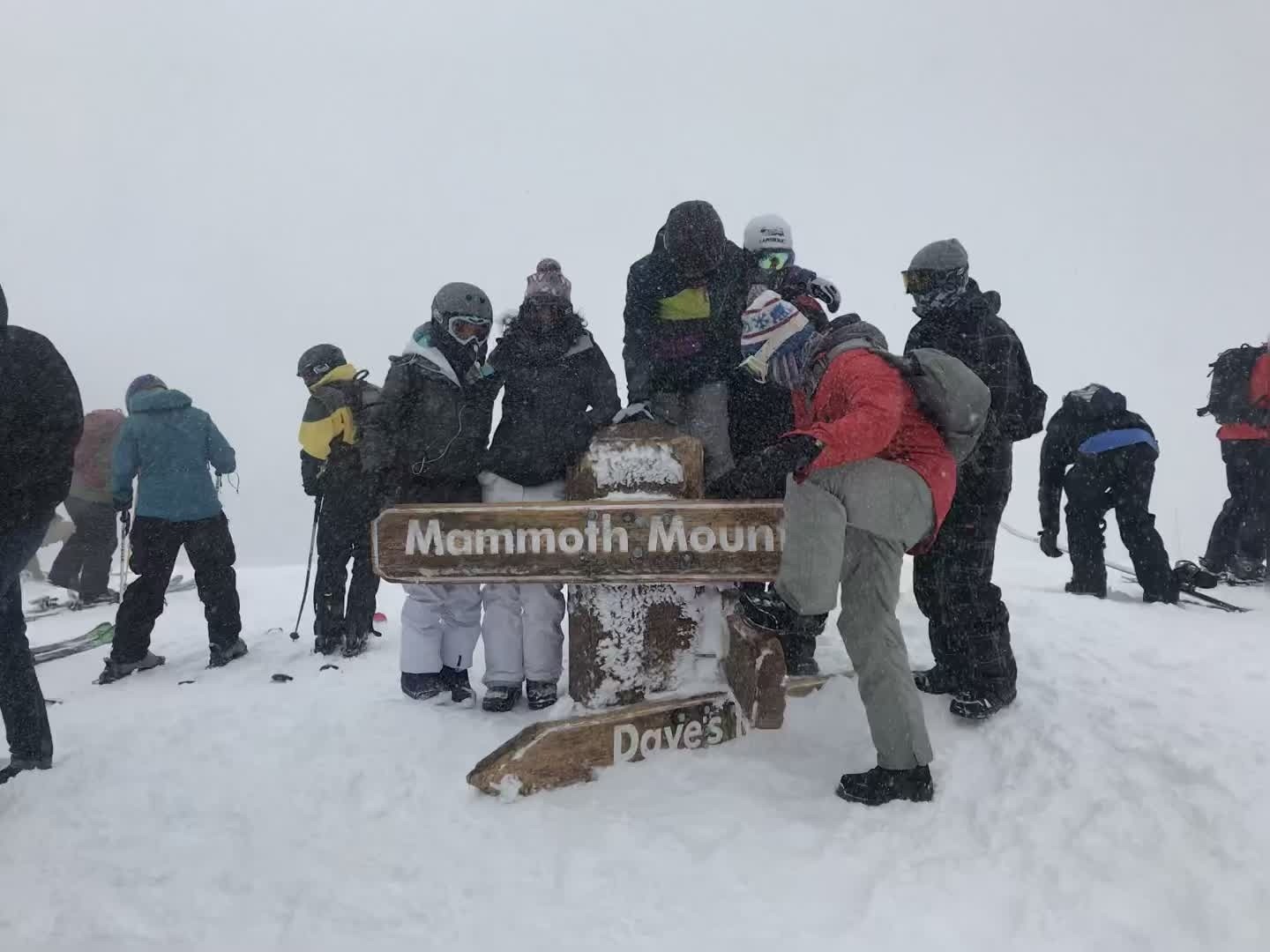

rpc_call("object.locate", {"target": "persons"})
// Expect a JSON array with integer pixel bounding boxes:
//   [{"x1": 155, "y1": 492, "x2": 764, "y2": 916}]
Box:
[
  {"x1": 1038, "y1": 409, "x2": 1179, "y2": 605},
  {"x1": 20, "y1": 513, "x2": 76, "y2": 581},
  {"x1": 903, "y1": 237, "x2": 1018, "y2": 718},
  {"x1": 103, "y1": 373, "x2": 247, "y2": 677},
  {"x1": 623, "y1": 199, "x2": 838, "y2": 500},
  {"x1": 297, "y1": 343, "x2": 380, "y2": 658},
  {"x1": 369, "y1": 282, "x2": 493, "y2": 705},
  {"x1": 0, "y1": 284, "x2": 85, "y2": 784},
  {"x1": 725, "y1": 213, "x2": 830, "y2": 676},
  {"x1": 477, "y1": 258, "x2": 621, "y2": 710},
  {"x1": 46, "y1": 408, "x2": 126, "y2": 602},
  {"x1": 1199, "y1": 332, "x2": 1270, "y2": 582},
  {"x1": 729, "y1": 283, "x2": 957, "y2": 806}
]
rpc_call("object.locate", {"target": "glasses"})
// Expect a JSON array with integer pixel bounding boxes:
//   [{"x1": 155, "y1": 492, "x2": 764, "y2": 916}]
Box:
[
  {"x1": 449, "y1": 316, "x2": 492, "y2": 347},
  {"x1": 739, "y1": 355, "x2": 768, "y2": 383},
  {"x1": 755, "y1": 249, "x2": 790, "y2": 270},
  {"x1": 901, "y1": 271, "x2": 914, "y2": 294}
]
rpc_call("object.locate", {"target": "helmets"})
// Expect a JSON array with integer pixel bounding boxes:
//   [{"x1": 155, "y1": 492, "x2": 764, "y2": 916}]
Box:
[
  {"x1": 126, "y1": 374, "x2": 168, "y2": 411},
  {"x1": 296, "y1": 344, "x2": 346, "y2": 376},
  {"x1": 431, "y1": 282, "x2": 493, "y2": 334}
]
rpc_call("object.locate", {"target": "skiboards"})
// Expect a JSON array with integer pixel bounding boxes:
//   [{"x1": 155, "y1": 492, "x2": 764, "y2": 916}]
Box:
[
  {"x1": 272, "y1": 612, "x2": 386, "y2": 681},
  {"x1": 24, "y1": 574, "x2": 201, "y2": 622},
  {"x1": 28, "y1": 622, "x2": 117, "y2": 665}
]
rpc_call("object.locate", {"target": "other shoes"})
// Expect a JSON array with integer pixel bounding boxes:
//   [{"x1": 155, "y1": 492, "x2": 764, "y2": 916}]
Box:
[{"x1": 0, "y1": 766, "x2": 21, "y2": 785}]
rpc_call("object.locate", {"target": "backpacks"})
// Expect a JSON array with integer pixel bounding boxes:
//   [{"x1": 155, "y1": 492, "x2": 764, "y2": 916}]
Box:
[
  {"x1": 1064, "y1": 383, "x2": 1126, "y2": 422},
  {"x1": 805, "y1": 338, "x2": 991, "y2": 463},
  {"x1": 325, "y1": 370, "x2": 381, "y2": 451},
  {"x1": 1197, "y1": 343, "x2": 1270, "y2": 427}
]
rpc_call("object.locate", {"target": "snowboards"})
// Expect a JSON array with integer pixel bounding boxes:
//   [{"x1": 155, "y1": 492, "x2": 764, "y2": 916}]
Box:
[{"x1": 999, "y1": 518, "x2": 1254, "y2": 613}]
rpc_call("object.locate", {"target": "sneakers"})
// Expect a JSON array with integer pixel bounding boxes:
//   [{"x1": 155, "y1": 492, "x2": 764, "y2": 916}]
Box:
[
  {"x1": 738, "y1": 588, "x2": 1018, "y2": 805},
  {"x1": 79, "y1": 588, "x2": 120, "y2": 603},
  {"x1": 209, "y1": 639, "x2": 247, "y2": 666},
  {"x1": 314, "y1": 631, "x2": 558, "y2": 713},
  {"x1": 53, "y1": 577, "x2": 78, "y2": 590},
  {"x1": 1065, "y1": 555, "x2": 1270, "y2": 605},
  {"x1": 110, "y1": 649, "x2": 162, "y2": 678}
]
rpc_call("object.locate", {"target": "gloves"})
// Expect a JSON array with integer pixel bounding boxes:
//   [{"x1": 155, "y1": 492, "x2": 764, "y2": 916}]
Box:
[
  {"x1": 760, "y1": 434, "x2": 823, "y2": 485},
  {"x1": 304, "y1": 479, "x2": 318, "y2": 496},
  {"x1": 1037, "y1": 528, "x2": 1063, "y2": 558},
  {"x1": 612, "y1": 401, "x2": 654, "y2": 423},
  {"x1": 806, "y1": 272, "x2": 841, "y2": 313}
]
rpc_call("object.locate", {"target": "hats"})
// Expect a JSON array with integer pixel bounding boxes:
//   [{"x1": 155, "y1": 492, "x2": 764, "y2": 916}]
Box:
[
  {"x1": 524, "y1": 258, "x2": 571, "y2": 303},
  {"x1": 664, "y1": 200, "x2": 969, "y2": 359}
]
